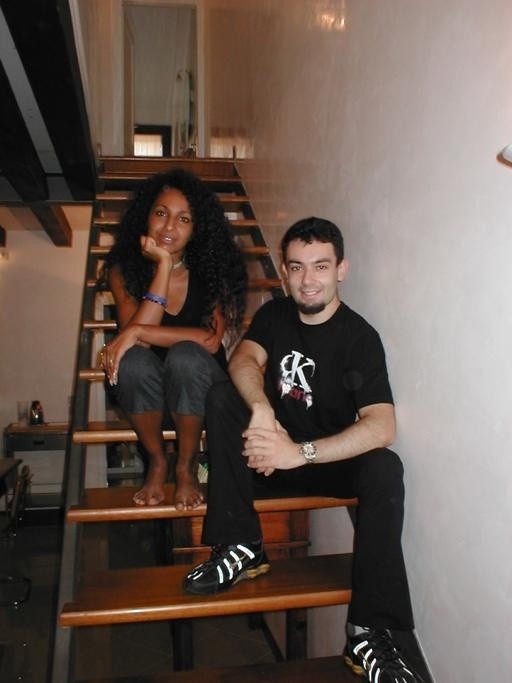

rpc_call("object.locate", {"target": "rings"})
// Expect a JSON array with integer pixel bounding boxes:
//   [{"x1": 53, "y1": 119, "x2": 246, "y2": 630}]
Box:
[
  {"x1": 109, "y1": 360, "x2": 114, "y2": 364},
  {"x1": 100, "y1": 352, "x2": 103, "y2": 356}
]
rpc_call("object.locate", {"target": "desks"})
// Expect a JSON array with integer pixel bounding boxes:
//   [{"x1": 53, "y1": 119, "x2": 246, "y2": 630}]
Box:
[{"x1": 3, "y1": 422, "x2": 68, "y2": 458}]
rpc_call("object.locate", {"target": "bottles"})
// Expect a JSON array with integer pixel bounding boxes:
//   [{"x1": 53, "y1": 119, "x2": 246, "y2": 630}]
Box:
[
  {"x1": 18, "y1": 400, "x2": 29, "y2": 426},
  {"x1": 30, "y1": 400, "x2": 43, "y2": 424}
]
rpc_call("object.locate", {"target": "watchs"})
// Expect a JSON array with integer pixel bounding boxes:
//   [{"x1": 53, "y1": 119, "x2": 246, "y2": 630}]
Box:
[{"x1": 299, "y1": 441, "x2": 316, "y2": 465}]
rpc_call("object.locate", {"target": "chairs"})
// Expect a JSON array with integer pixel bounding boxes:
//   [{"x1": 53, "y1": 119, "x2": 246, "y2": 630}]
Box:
[{"x1": 0, "y1": 464, "x2": 35, "y2": 610}]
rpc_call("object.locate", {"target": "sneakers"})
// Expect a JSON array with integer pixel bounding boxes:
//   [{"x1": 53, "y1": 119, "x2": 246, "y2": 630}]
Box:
[
  {"x1": 182, "y1": 537, "x2": 270, "y2": 595},
  {"x1": 344, "y1": 623, "x2": 424, "y2": 683}
]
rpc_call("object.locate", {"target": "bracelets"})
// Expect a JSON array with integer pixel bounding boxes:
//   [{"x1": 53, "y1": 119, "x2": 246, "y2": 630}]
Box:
[{"x1": 143, "y1": 292, "x2": 167, "y2": 308}]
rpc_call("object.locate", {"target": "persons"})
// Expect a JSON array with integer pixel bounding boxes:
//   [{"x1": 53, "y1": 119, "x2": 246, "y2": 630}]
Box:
[
  {"x1": 183, "y1": 217, "x2": 426, "y2": 682},
  {"x1": 93, "y1": 168, "x2": 245, "y2": 510}
]
